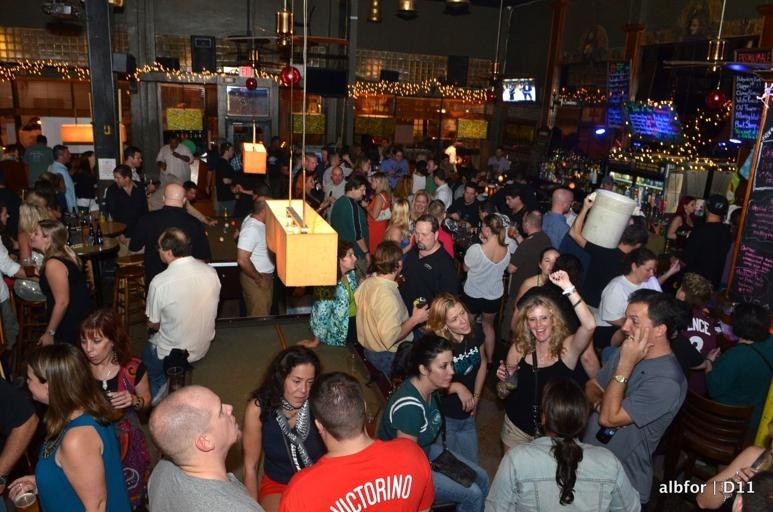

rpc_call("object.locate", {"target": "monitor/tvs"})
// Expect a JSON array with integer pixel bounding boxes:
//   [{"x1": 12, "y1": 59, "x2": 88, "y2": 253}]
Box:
[
  {"x1": 500, "y1": 74, "x2": 539, "y2": 105},
  {"x1": 224, "y1": 83, "x2": 272, "y2": 120}
]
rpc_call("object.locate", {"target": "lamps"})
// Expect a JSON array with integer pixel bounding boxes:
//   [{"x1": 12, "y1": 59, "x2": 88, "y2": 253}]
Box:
[
  {"x1": 246, "y1": 66, "x2": 258, "y2": 92},
  {"x1": 240, "y1": 118, "x2": 268, "y2": 175},
  {"x1": 61, "y1": 124, "x2": 127, "y2": 145},
  {"x1": 281, "y1": 66, "x2": 301, "y2": 87},
  {"x1": 265, "y1": 0, "x2": 338, "y2": 287}
]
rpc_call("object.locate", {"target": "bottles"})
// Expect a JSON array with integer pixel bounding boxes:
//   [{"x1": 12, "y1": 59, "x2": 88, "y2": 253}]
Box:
[
  {"x1": 62, "y1": 208, "x2": 103, "y2": 245},
  {"x1": 414, "y1": 296, "x2": 426, "y2": 327}
]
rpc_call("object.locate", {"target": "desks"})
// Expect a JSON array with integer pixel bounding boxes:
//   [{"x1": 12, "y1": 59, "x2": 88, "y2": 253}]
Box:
[{"x1": 204, "y1": 217, "x2": 247, "y2": 318}]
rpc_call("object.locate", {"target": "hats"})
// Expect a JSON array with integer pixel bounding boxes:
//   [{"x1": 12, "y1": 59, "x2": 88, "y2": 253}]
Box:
[{"x1": 706, "y1": 194, "x2": 729, "y2": 215}]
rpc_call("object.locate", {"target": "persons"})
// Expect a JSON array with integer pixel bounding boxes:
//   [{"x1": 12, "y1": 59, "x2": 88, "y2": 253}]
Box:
[{"x1": 0, "y1": 133, "x2": 772, "y2": 512}]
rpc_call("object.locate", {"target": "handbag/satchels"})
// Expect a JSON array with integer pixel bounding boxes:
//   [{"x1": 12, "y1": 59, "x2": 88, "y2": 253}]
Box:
[
  {"x1": 375, "y1": 207, "x2": 392, "y2": 221},
  {"x1": 430, "y1": 449, "x2": 477, "y2": 488}
]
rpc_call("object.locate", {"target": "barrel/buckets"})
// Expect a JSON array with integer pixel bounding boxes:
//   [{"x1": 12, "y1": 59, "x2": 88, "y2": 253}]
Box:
[{"x1": 580, "y1": 190, "x2": 635, "y2": 251}]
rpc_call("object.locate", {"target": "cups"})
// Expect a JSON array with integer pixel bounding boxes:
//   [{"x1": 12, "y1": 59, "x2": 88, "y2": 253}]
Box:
[
  {"x1": 508, "y1": 221, "x2": 517, "y2": 239},
  {"x1": 504, "y1": 360, "x2": 517, "y2": 389},
  {"x1": 23, "y1": 257, "x2": 36, "y2": 277},
  {"x1": 9, "y1": 481, "x2": 39, "y2": 511},
  {"x1": 103, "y1": 390, "x2": 122, "y2": 420}
]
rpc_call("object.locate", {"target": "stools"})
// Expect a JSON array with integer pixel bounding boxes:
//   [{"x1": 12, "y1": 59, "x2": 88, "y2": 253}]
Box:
[
  {"x1": 13, "y1": 279, "x2": 48, "y2": 378},
  {"x1": 113, "y1": 255, "x2": 146, "y2": 339}
]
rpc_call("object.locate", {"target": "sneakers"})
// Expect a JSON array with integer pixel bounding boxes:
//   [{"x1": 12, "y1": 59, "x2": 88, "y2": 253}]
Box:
[{"x1": 13, "y1": 377, "x2": 25, "y2": 390}]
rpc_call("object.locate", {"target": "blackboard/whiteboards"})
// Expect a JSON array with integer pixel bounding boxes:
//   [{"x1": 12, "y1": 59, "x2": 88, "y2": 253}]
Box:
[
  {"x1": 728, "y1": 96, "x2": 773, "y2": 309},
  {"x1": 605, "y1": 58, "x2": 632, "y2": 128},
  {"x1": 625, "y1": 100, "x2": 682, "y2": 143},
  {"x1": 728, "y1": 49, "x2": 773, "y2": 141}
]
